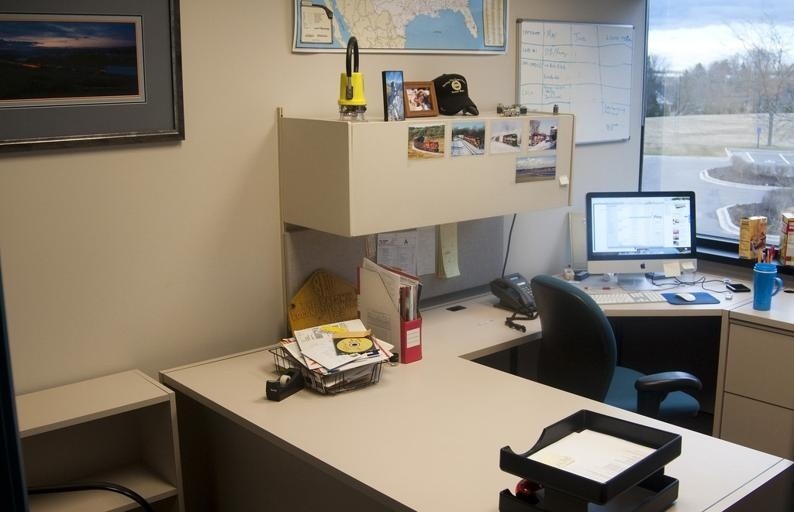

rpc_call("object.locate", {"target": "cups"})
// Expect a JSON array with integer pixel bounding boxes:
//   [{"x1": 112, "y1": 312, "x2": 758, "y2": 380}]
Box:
[{"x1": 752, "y1": 261, "x2": 783, "y2": 310}]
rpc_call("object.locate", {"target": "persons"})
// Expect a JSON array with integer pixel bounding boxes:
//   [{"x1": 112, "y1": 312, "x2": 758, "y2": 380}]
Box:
[{"x1": 387, "y1": 80, "x2": 404, "y2": 120}]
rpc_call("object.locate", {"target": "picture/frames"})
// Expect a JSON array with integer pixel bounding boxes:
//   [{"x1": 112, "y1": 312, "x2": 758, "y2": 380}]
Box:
[
  {"x1": 403, "y1": 80, "x2": 439, "y2": 119},
  {"x1": 0, "y1": 0, "x2": 185, "y2": 158}
]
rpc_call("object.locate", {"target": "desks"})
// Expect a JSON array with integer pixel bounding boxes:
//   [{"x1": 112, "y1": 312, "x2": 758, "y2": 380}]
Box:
[{"x1": 153, "y1": 260, "x2": 792, "y2": 509}]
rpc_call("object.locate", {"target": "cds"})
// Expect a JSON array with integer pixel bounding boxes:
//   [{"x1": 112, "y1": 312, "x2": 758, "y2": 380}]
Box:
[{"x1": 337, "y1": 337, "x2": 373, "y2": 353}]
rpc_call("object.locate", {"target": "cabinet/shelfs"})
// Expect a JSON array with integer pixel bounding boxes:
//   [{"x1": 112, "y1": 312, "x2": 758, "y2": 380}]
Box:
[{"x1": 11, "y1": 369, "x2": 185, "y2": 509}]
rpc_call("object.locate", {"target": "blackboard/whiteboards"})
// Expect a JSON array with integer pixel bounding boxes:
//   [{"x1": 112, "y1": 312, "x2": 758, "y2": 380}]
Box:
[{"x1": 516, "y1": 18, "x2": 635, "y2": 146}]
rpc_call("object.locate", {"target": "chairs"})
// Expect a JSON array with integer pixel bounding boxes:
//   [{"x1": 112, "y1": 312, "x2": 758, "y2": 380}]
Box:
[{"x1": 527, "y1": 274, "x2": 704, "y2": 424}]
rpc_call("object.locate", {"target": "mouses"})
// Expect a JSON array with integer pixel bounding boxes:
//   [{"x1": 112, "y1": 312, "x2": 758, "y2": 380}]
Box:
[{"x1": 676, "y1": 292, "x2": 695, "y2": 301}]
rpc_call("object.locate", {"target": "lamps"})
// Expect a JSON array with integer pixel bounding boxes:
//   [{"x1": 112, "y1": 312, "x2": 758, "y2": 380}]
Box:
[{"x1": 340, "y1": 37, "x2": 371, "y2": 122}]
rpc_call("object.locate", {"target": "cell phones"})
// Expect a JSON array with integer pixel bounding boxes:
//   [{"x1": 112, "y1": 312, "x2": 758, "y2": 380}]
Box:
[{"x1": 727, "y1": 283, "x2": 751, "y2": 292}]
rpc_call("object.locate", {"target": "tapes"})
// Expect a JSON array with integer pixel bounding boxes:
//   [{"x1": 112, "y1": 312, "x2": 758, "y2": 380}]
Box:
[{"x1": 280, "y1": 374, "x2": 291, "y2": 385}]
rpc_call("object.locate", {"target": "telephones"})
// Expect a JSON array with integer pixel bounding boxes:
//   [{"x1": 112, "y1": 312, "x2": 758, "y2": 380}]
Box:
[{"x1": 490, "y1": 273, "x2": 536, "y2": 314}]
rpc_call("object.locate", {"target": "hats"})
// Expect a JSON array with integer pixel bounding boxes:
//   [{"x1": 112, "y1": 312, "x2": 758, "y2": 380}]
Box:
[{"x1": 431, "y1": 74, "x2": 479, "y2": 115}]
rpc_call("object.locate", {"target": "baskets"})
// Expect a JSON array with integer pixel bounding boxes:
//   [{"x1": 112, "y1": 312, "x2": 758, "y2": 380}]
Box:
[{"x1": 266, "y1": 346, "x2": 382, "y2": 396}]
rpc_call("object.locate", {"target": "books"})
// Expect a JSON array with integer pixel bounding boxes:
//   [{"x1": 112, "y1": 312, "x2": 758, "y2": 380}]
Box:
[
  {"x1": 357, "y1": 257, "x2": 422, "y2": 364},
  {"x1": 280, "y1": 317, "x2": 395, "y2": 390}
]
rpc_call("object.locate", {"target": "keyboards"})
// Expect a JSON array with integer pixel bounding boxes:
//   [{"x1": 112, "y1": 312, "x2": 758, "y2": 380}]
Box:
[{"x1": 587, "y1": 291, "x2": 667, "y2": 305}]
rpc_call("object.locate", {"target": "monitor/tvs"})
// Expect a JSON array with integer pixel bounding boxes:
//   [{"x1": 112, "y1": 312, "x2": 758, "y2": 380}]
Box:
[{"x1": 587, "y1": 191, "x2": 698, "y2": 292}]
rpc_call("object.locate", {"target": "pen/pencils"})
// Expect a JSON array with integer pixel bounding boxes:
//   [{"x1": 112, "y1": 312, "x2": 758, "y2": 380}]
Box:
[
  {"x1": 584, "y1": 286, "x2": 612, "y2": 290},
  {"x1": 757, "y1": 244, "x2": 775, "y2": 264}
]
